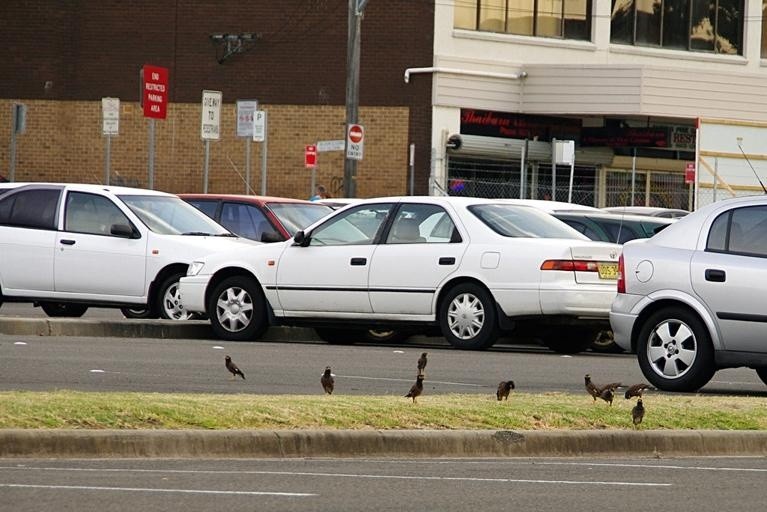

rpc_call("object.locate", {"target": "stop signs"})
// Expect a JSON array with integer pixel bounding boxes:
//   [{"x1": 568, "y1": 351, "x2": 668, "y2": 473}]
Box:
[{"x1": 349, "y1": 126, "x2": 362, "y2": 143}]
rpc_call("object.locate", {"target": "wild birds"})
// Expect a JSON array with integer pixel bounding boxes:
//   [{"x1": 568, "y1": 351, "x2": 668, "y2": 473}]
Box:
[
  {"x1": 585, "y1": 374, "x2": 628, "y2": 407},
  {"x1": 497, "y1": 381, "x2": 515, "y2": 401},
  {"x1": 224, "y1": 355, "x2": 246, "y2": 382},
  {"x1": 625, "y1": 383, "x2": 659, "y2": 400},
  {"x1": 633, "y1": 399, "x2": 644, "y2": 425},
  {"x1": 320, "y1": 366, "x2": 334, "y2": 395},
  {"x1": 417, "y1": 353, "x2": 427, "y2": 375},
  {"x1": 405, "y1": 375, "x2": 424, "y2": 404}
]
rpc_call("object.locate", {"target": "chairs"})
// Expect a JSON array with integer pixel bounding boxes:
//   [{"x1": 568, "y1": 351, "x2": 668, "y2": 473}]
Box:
[{"x1": 395, "y1": 218, "x2": 426, "y2": 243}]
